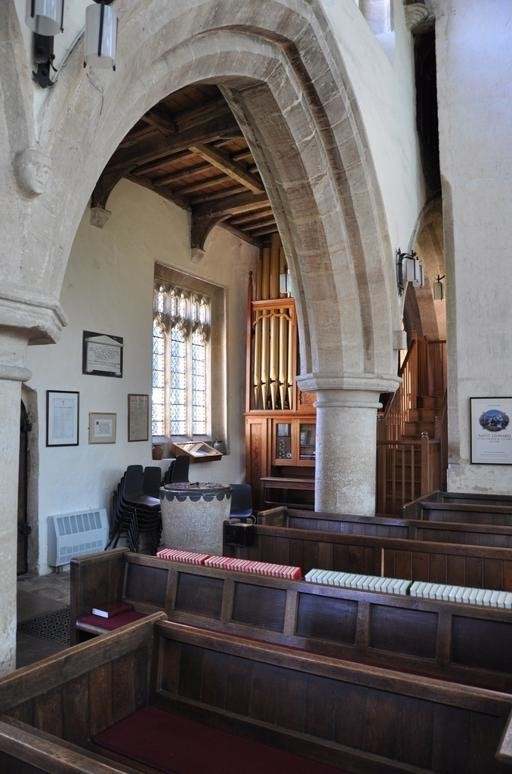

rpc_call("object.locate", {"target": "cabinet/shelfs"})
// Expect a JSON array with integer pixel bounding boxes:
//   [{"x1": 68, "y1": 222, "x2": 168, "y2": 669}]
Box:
[{"x1": 244, "y1": 410, "x2": 319, "y2": 512}]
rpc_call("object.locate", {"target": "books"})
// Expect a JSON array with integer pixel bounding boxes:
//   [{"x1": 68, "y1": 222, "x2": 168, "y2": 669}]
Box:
[{"x1": 91, "y1": 600, "x2": 132, "y2": 619}]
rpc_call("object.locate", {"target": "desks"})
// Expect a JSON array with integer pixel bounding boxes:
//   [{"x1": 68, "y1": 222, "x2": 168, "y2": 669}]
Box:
[{"x1": 259, "y1": 477, "x2": 314, "y2": 511}]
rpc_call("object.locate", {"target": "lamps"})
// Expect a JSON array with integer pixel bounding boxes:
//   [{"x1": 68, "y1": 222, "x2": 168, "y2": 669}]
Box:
[
  {"x1": 397, "y1": 249, "x2": 425, "y2": 293},
  {"x1": 22, "y1": 0, "x2": 121, "y2": 89},
  {"x1": 431, "y1": 276, "x2": 445, "y2": 300}
]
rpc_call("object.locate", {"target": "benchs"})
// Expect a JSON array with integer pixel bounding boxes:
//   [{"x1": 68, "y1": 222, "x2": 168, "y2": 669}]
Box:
[
  {"x1": 1, "y1": 610, "x2": 512, "y2": 774},
  {"x1": 222, "y1": 506, "x2": 512, "y2": 593},
  {"x1": 70, "y1": 547, "x2": 512, "y2": 694},
  {"x1": 402, "y1": 489, "x2": 512, "y2": 526}
]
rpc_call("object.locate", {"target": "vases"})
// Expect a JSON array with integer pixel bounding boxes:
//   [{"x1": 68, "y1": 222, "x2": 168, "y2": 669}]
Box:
[
  {"x1": 152, "y1": 445, "x2": 164, "y2": 460},
  {"x1": 215, "y1": 440, "x2": 226, "y2": 454}
]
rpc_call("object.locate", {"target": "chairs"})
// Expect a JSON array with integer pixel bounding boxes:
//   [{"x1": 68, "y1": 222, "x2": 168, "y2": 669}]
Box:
[
  {"x1": 106, "y1": 456, "x2": 190, "y2": 555},
  {"x1": 229, "y1": 484, "x2": 256, "y2": 524}
]
